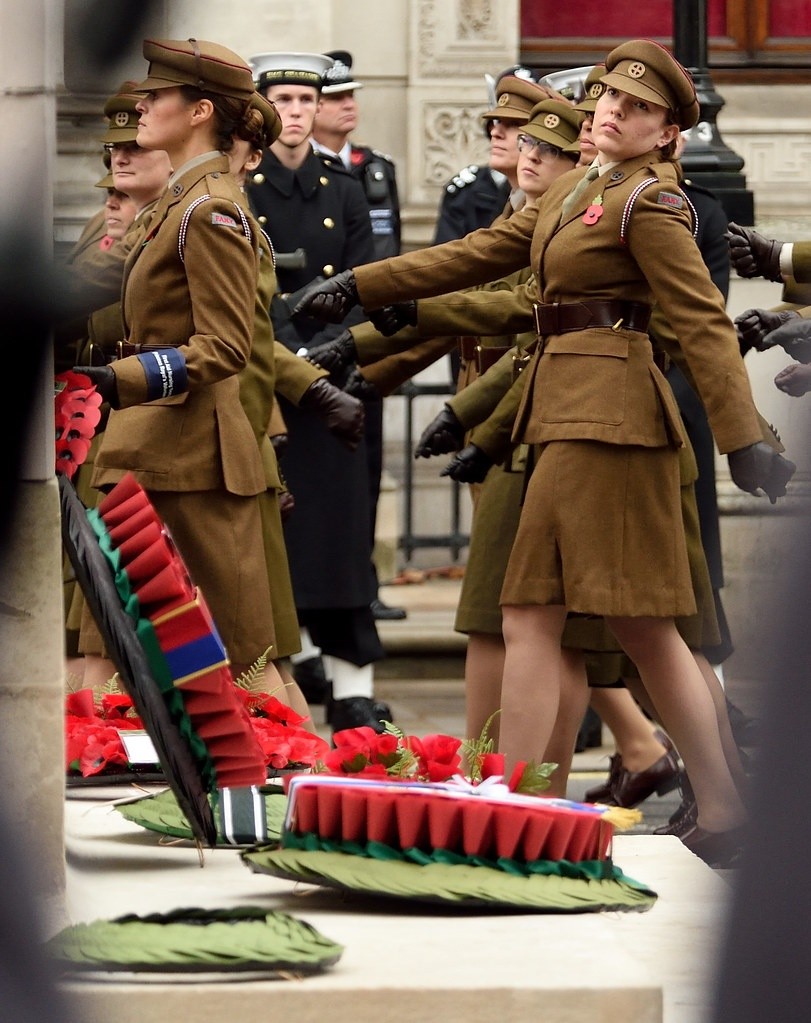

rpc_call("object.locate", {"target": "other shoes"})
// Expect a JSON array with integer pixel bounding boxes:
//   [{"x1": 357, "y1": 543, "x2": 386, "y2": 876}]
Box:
[
  {"x1": 327, "y1": 699, "x2": 393, "y2": 724},
  {"x1": 295, "y1": 655, "x2": 326, "y2": 702},
  {"x1": 576, "y1": 709, "x2": 603, "y2": 752},
  {"x1": 370, "y1": 599, "x2": 405, "y2": 620},
  {"x1": 337, "y1": 697, "x2": 384, "y2": 745}
]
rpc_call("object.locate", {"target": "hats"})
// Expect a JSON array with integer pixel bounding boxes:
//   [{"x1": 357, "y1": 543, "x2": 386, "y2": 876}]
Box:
[
  {"x1": 323, "y1": 50, "x2": 362, "y2": 95},
  {"x1": 572, "y1": 66, "x2": 607, "y2": 112},
  {"x1": 482, "y1": 75, "x2": 550, "y2": 120},
  {"x1": 101, "y1": 95, "x2": 143, "y2": 143},
  {"x1": 496, "y1": 64, "x2": 540, "y2": 84},
  {"x1": 252, "y1": 91, "x2": 283, "y2": 147},
  {"x1": 598, "y1": 40, "x2": 699, "y2": 132},
  {"x1": 132, "y1": 37, "x2": 254, "y2": 100},
  {"x1": 249, "y1": 52, "x2": 335, "y2": 90},
  {"x1": 94, "y1": 154, "x2": 115, "y2": 188},
  {"x1": 519, "y1": 99, "x2": 585, "y2": 148}
]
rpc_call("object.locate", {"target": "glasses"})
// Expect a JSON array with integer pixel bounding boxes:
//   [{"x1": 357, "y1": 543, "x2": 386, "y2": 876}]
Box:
[
  {"x1": 517, "y1": 134, "x2": 559, "y2": 163},
  {"x1": 104, "y1": 142, "x2": 141, "y2": 155}
]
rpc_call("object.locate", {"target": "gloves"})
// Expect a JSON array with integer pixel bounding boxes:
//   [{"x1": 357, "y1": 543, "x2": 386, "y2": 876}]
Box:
[
  {"x1": 722, "y1": 221, "x2": 779, "y2": 281},
  {"x1": 293, "y1": 269, "x2": 355, "y2": 324},
  {"x1": 305, "y1": 330, "x2": 358, "y2": 371},
  {"x1": 439, "y1": 445, "x2": 491, "y2": 485},
  {"x1": 727, "y1": 441, "x2": 797, "y2": 505},
  {"x1": 73, "y1": 365, "x2": 116, "y2": 402},
  {"x1": 306, "y1": 378, "x2": 366, "y2": 454},
  {"x1": 415, "y1": 412, "x2": 464, "y2": 459},
  {"x1": 735, "y1": 308, "x2": 811, "y2": 363},
  {"x1": 774, "y1": 363, "x2": 811, "y2": 398}
]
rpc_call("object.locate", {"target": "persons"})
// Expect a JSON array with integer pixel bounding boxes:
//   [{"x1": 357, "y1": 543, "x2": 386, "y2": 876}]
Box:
[
  {"x1": 54, "y1": 36, "x2": 811, "y2": 837},
  {"x1": 292, "y1": 40, "x2": 795, "y2": 867}
]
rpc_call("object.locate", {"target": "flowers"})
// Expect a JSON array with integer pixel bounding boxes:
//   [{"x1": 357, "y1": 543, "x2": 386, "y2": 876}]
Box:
[
  {"x1": 55, "y1": 372, "x2": 642, "y2": 878},
  {"x1": 582, "y1": 194, "x2": 603, "y2": 224},
  {"x1": 100, "y1": 236, "x2": 113, "y2": 251}
]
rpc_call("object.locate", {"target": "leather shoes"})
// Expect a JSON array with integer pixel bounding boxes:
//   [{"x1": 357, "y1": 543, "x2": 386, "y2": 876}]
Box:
[
  {"x1": 585, "y1": 753, "x2": 682, "y2": 807},
  {"x1": 610, "y1": 731, "x2": 681, "y2": 779},
  {"x1": 655, "y1": 802, "x2": 698, "y2": 835},
  {"x1": 682, "y1": 821, "x2": 754, "y2": 864}
]
